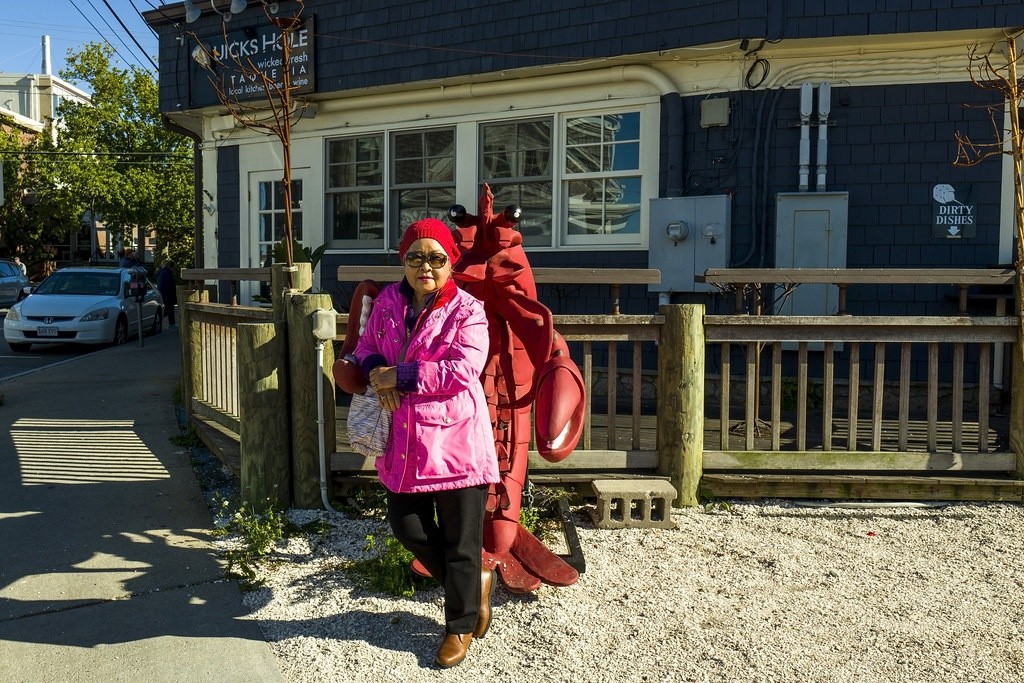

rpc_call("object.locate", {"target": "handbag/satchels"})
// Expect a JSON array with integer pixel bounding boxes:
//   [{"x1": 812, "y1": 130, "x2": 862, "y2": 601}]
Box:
[{"x1": 347, "y1": 392, "x2": 391, "y2": 457}]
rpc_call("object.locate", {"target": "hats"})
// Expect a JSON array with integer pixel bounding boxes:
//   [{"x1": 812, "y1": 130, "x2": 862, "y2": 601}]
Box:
[{"x1": 398, "y1": 217, "x2": 460, "y2": 264}]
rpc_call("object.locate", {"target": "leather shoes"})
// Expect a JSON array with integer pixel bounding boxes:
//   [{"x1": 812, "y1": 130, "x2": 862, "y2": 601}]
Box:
[
  {"x1": 435, "y1": 630, "x2": 473, "y2": 668},
  {"x1": 474, "y1": 568, "x2": 497, "y2": 639}
]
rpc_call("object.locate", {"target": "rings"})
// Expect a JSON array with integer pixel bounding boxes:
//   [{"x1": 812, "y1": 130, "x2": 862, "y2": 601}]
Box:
[
  {"x1": 375, "y1": 386, "x2": 378, "y2": 391},
  {"x1": 380, "y1": 396, "x2": 386, "y2": 401},
  {"x1": 373, "y1": 382, "x2": 375, "y2": 386}
]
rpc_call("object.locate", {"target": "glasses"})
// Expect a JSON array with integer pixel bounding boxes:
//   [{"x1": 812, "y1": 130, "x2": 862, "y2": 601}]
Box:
[{"x1": 406, "y1": 252, "x2": 448, "y2": 267}]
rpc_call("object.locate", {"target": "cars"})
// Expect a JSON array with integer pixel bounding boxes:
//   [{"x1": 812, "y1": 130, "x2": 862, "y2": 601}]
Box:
[
  {"x1": 0, "y1": 261, "x2": 28, "y2": 309},
  {"x1": 4, "y1": 266, "x2": 165, "y2": 353}
]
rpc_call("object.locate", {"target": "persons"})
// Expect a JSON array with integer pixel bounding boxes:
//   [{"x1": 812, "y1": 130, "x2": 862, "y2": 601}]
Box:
[
  {"x1": 14, "y1": 257, "x2": 26, "y2": 276},
  {"x1": 120, "y1": 248, "x2": 143, "y2": 268},
  {"x1": 354, "y1": 219, "x2": 496, "y2": 669},
  {"x1": 44, "y1": 259, "x2": 56, "y2": 276},
  {"x1": 156, "y1": 262, "x2": 179, "y2": 329}
]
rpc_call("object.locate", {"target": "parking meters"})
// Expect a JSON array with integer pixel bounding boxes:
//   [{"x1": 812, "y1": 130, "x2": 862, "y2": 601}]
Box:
[{"x1": 127, "y1": 266, "x2": 148, "y2": 348}]
